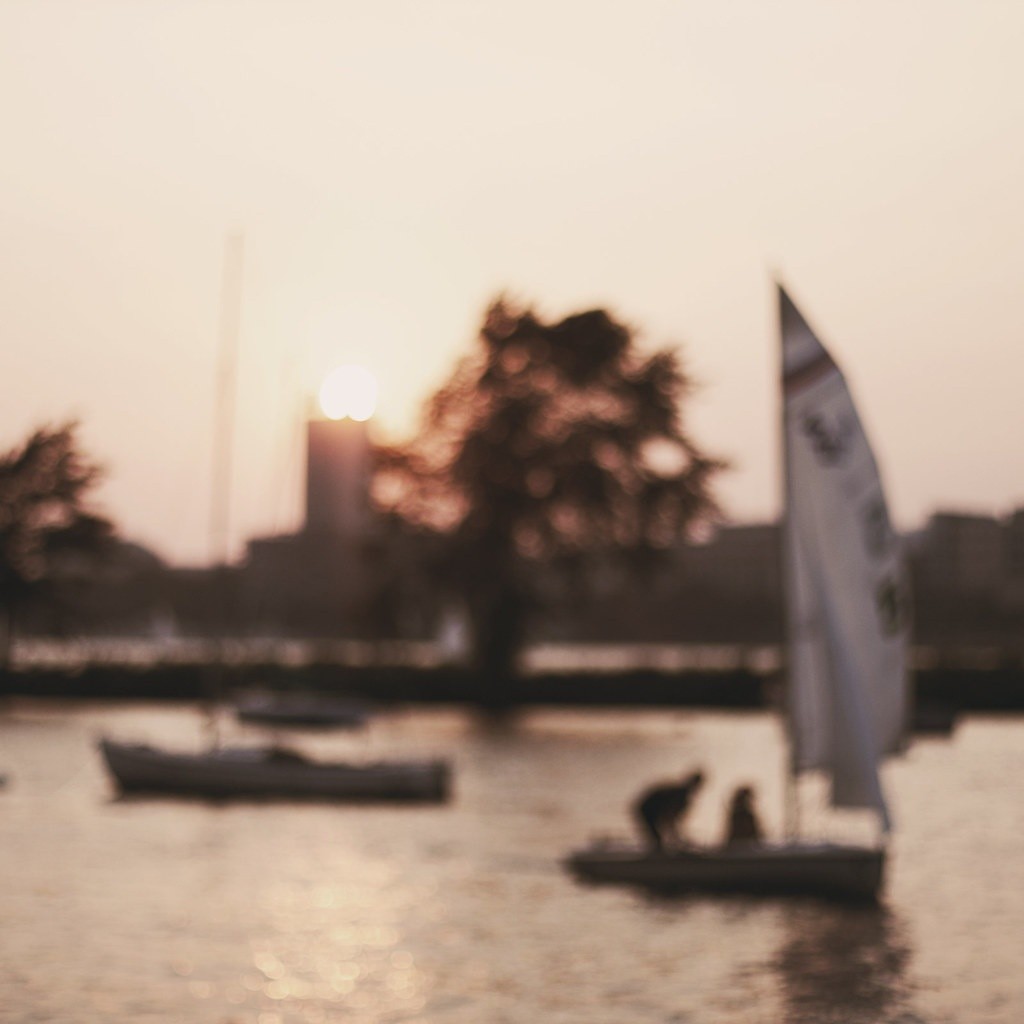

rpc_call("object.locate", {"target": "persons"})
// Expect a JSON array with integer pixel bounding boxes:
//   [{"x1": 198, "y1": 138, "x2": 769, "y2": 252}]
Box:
[
  {"x1": 724, "y1": 786, "x2": 763, "y2": 846},
  {"x1": 628, "y1": 772, "x2": 702, "y2": 857}
]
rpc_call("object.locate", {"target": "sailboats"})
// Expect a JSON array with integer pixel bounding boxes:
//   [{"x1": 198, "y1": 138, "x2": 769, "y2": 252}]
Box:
[{"x1": 561, "y1": 286, "x2": 909, "y2": 901}]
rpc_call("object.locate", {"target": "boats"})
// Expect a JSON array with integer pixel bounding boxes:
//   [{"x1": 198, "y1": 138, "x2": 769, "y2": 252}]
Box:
[
  {"x1": 98, "y1": 734, "x2": 454, "y2": 805},
  {"x1": 239, "y1": 692, "x2": 366, "y2": 725}
]
